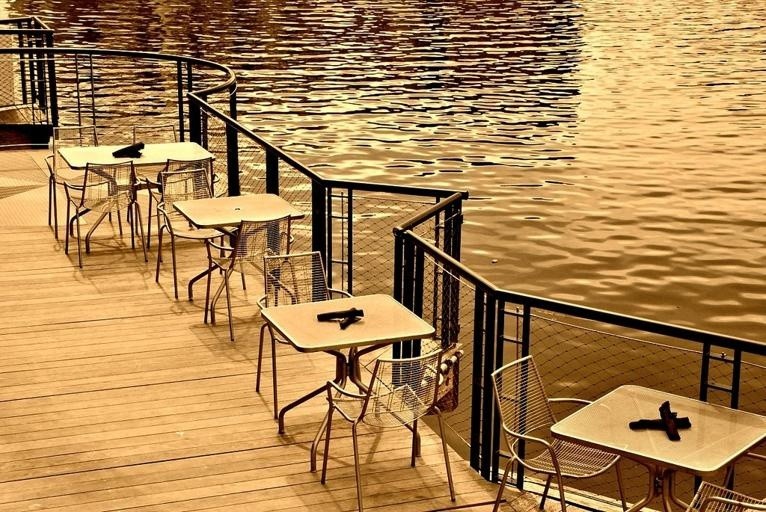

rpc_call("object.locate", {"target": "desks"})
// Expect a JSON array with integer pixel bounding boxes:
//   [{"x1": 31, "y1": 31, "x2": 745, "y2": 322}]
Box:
[
  {"x1": 171, "y1": 191, "x2": 305, "y2": 326},
  {"x1": 549, "y1": 381, "x2": 766, "y2": 512},
  {"x1": 259, "y1": 292, "x2": 439, "y2": 472},
  {"x1": 57, "y1": 141, "x2": 217, "y2": 255}
]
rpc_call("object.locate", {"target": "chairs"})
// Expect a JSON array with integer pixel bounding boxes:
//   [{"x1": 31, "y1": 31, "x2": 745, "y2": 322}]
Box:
[
  {"x1": 125, "y1": 124, "x2": 191, "y2": 237},
  {"x1": 681, "y1": 452, "x2": 765, "y2": 512},
  {"x1": 62, "y1": 160, "x2": 149, "y2": 269},
  {"x1": 154, "y1": 168, "x2": 247, "y2": 299},
  {"x1": 318, "y1": 347, "x2": 456, "y2": 511},
  {"x1": 254, "y1": 249, "x2": 365, "y2": 421},
  {"x1": 145, "y1": 155, "x2": 215, "y2": 264},
  {"x1": 43, "y1": 123, "x2": 125, "y2": 241},
  {"x1": 203, "y1": 213, "x2": 300, "y2": 342},
  {"x1": 489, "y1": 352, "x2": 628, "y2": 512}
]
